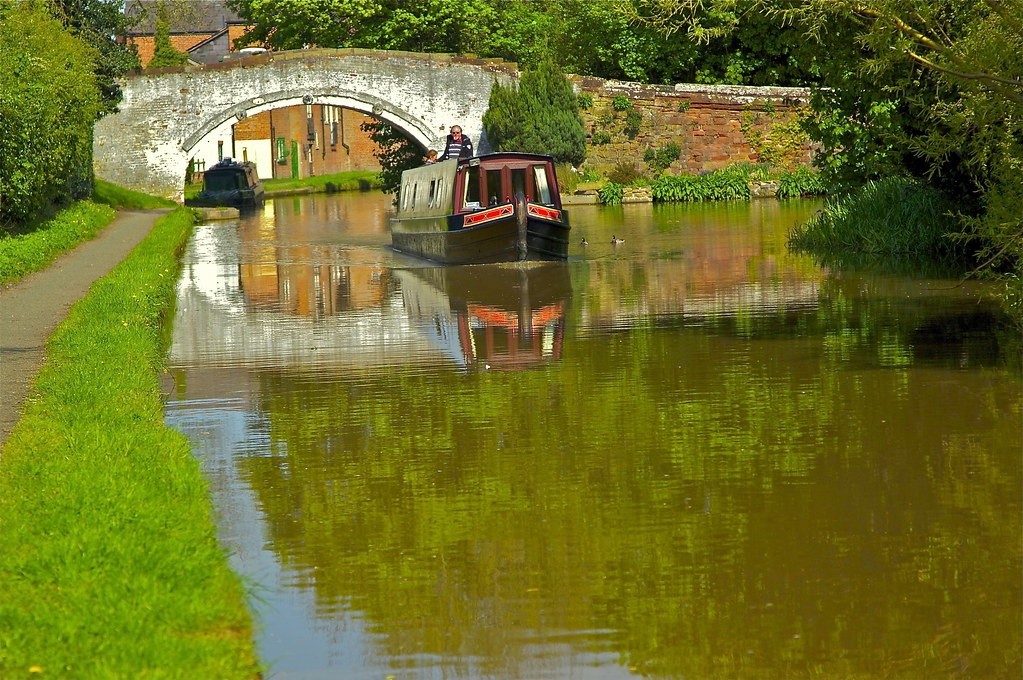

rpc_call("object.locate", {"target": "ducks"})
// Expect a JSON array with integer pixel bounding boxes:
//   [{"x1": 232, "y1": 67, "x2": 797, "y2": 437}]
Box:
[
  {"x1": 581, "y1": 237, "x2": 588, "y2": 245},
  {"x1": 612, "y1": 234, "x2": 625, "y2": 244}
]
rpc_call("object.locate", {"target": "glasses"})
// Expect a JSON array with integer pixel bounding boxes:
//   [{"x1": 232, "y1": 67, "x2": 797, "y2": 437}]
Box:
[{"x1": 452, "y1": 132, "x2": 459, "y2": 135}]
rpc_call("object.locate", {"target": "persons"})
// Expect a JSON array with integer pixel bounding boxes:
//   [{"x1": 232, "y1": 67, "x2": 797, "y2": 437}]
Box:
[
  {"x1": 438, "y1": 125, "x2": 473, "y2": 161},
  {"x1": 422, "y1": 150, "x2": 438, "y2": 165}
]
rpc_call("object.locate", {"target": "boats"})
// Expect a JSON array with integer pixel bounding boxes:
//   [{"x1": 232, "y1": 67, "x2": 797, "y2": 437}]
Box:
[
  {"x1": 195, "y1": 160, "x2": 265, "y2": 211},
  {"x1": 388, "y1": 153, "x2": 573, "y2": 264}
]
